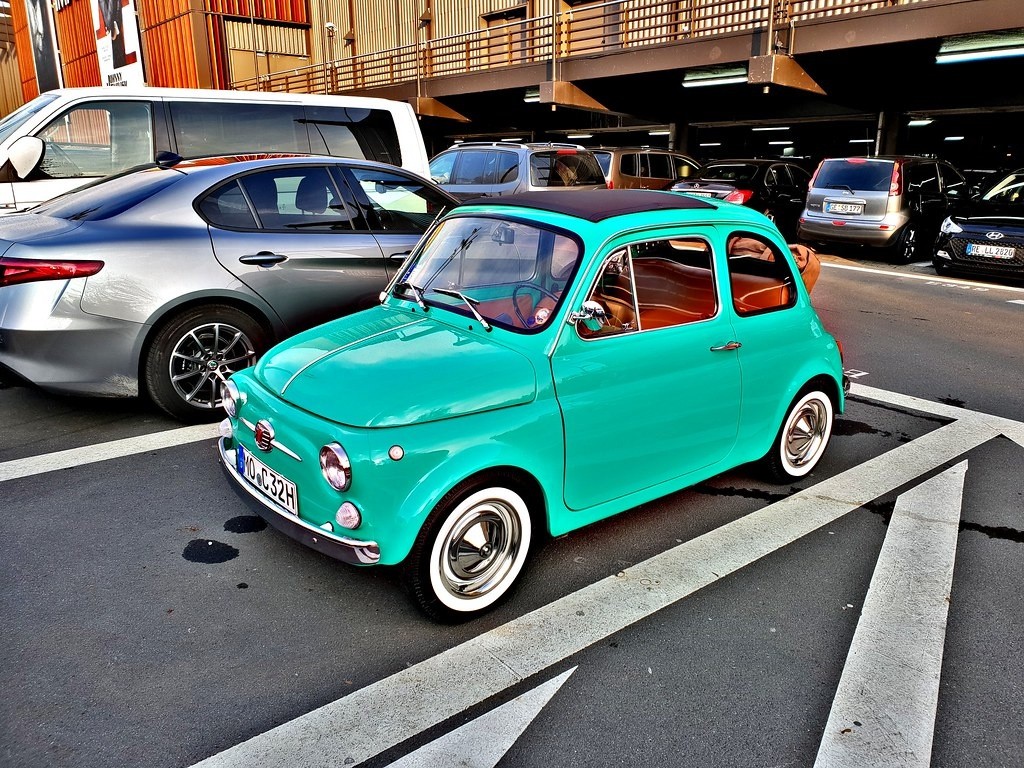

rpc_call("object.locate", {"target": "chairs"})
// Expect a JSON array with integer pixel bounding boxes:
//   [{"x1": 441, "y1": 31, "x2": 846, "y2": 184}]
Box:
[
  {"x1": 294, "y1": 177, "x2": 334, "y2": 232},
  {"x1": 242, "y1": 179, "x2": 283, "y2": 229},
  {"x1": 532, "y1": 292, "x2": 638, "y2": 337}
]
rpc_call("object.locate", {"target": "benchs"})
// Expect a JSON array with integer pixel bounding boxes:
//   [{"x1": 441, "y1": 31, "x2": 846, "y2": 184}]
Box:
[{"x1": 622, "y1": 257, "x2": 790, "y2": 329}]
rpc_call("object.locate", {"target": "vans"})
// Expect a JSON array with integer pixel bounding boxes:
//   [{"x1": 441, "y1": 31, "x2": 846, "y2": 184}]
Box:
[{"x1": 0, "y1": 85, "x2": 432, "y2": 221}]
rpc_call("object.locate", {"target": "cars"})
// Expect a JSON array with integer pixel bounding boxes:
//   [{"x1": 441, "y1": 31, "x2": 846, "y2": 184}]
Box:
[
  {"x1": 215, "y1": 186, "x2": 850, "y2": 628},
  {"x1": 931, "y1": 165, "x2": 1024, "y2": 286},
  {"x1": 660, "y1": 155, "x2": 813, "y2": 244},
  {"x1": 0, "y1": 159, "x2": 487, "y2": 424}
]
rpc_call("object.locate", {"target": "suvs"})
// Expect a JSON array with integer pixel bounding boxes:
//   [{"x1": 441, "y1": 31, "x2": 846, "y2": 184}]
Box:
[
  {"x1": 797, "y1": 152, "x2": 975, "y2": 264},
  {"x1": 427, "y1": 138, "x2": 609, "y2": 203},
  {"x1": 583, "y1": 143, "x2": 702, "y2": 189}
]
